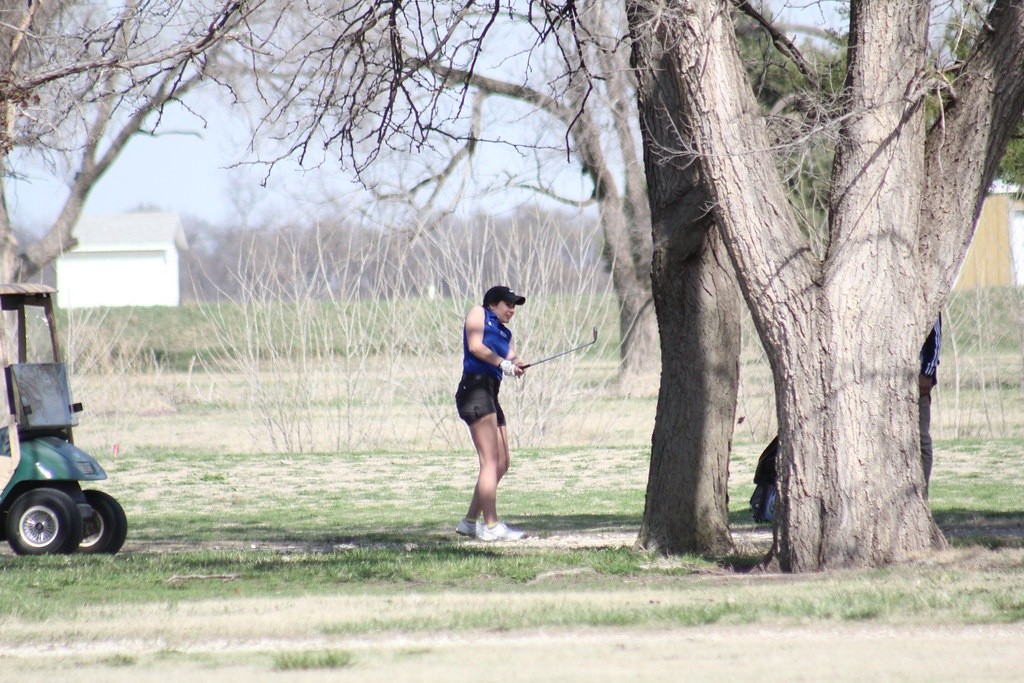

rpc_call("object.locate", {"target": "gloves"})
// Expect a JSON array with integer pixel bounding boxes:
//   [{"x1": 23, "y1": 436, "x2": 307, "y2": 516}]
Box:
[{"x1": 500, "y1": 359, "x2": 517, "y2": 376}]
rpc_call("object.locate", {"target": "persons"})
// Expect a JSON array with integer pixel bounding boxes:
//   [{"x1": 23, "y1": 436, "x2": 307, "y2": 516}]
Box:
[
  {"x1": 918, "y1": 311, "x2": 942, "y2": 503},
  {"x1": 452, "y1": 286, "x2": 529, "y2": 541},
  {"x1": 750, "y1": 435, "x2": 779, "y2": 522}
]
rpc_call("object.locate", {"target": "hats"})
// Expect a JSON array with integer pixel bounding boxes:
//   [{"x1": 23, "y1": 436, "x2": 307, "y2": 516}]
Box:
[{"x1": 483, "y1": 285, "x2": 525, "y2": 307}]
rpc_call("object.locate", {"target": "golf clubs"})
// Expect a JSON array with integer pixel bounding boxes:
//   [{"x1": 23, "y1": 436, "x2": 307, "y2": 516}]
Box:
[{"x1": 520, "y1": 327, "x2": 597, "y2": 369}]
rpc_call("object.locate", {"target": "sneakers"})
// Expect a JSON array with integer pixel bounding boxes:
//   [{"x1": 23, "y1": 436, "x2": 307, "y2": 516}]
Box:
[{"x1": 454, "y1": 518, "x2": 526, "y2": 541}]
